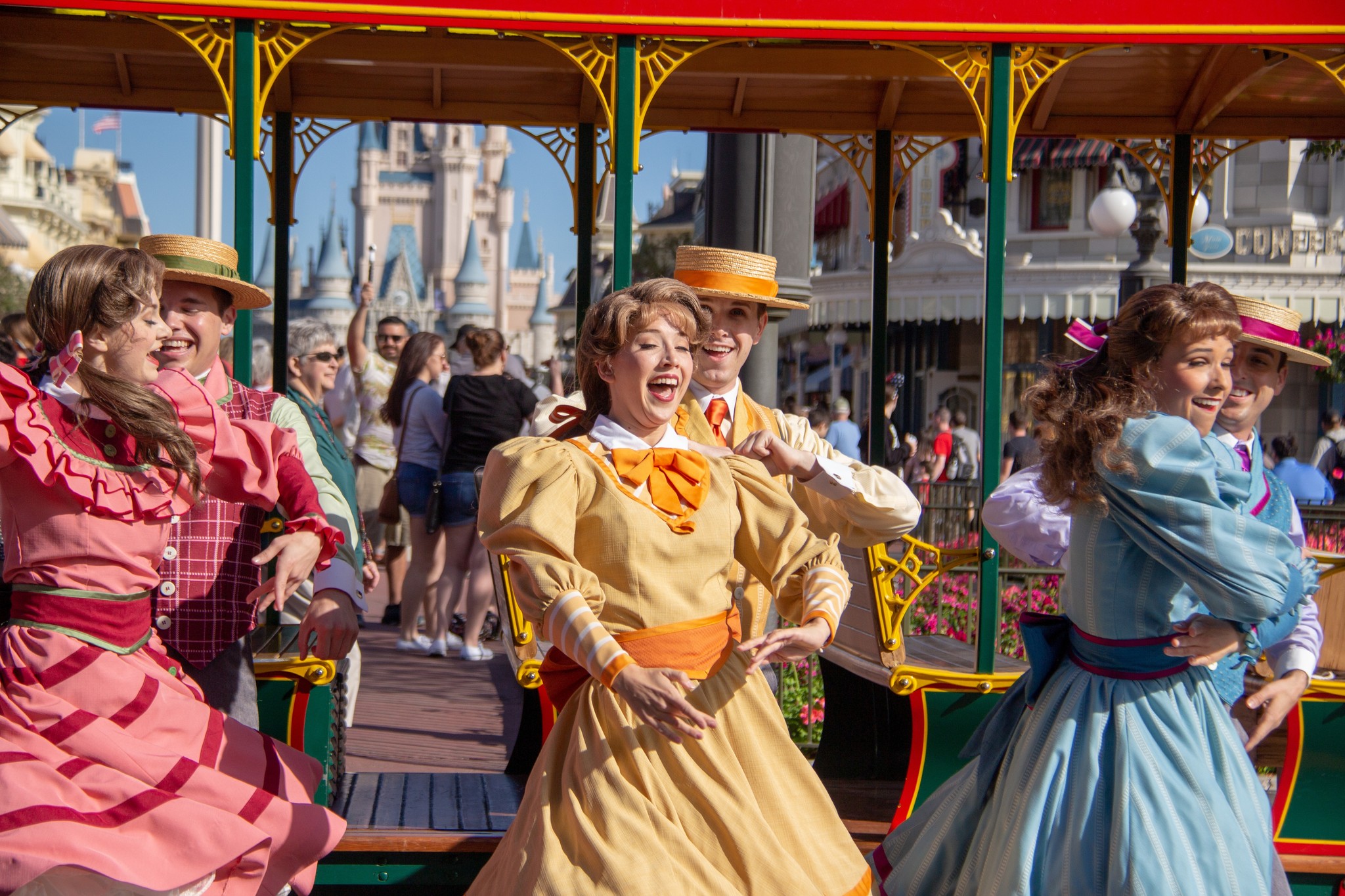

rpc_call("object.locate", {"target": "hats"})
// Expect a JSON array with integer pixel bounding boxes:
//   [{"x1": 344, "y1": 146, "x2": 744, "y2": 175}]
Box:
[
  {"x1": 674, "y1": 245, "x2": 810, "y2": 310},
  {"x1": 140, "y1": 235, "x2": 272, "y2": 309},
  {"x1": 1229, "y1": 294, "x2": 1333, "y2": 366}
]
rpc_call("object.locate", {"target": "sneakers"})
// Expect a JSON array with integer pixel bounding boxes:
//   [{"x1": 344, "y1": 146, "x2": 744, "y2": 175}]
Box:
[
  {"x1": 432, "y1": 630, "x2": 463, "y2": 651},
  {"x1": 396, "y1": 634, "x2": 432, "y2": 653},
  {"x1": 460, "y1": 641, "x2": 493, "y2": 661},
  {"x1": 427, "y1": 639, "x2": 450, "y2": 656}
]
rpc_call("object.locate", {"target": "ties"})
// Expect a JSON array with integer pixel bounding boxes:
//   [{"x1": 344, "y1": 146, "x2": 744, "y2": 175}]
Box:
[
  {"x1": 1234, "y1": 448, "x2": 1254, "y2": 473},
  {"x1": 704, "y1": 398, "x2": 727, "y2": 447}
]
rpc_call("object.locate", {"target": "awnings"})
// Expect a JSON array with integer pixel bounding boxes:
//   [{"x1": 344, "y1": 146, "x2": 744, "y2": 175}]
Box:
[{"x1": 1012, "y1": 137, "x2": 1211, "y2": 170}]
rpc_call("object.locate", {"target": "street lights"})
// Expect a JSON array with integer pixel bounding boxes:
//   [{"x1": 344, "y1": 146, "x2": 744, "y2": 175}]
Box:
[{"x1": 1088, "y1": 135, "x2": 1209, "y2": 320}]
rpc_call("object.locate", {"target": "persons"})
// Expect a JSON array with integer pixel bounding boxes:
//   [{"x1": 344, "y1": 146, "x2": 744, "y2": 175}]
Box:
[
  {"x1": 220, "y1": 337, "x2": 272, "y2": 392},
  {"x1": 287, "y1": 312, "x2": 378, "y2": 727},
  {"x1": 530, "y1": 244, "x2": 921, "y2": 696},
  {"x1": 981, "y1": 298, "x2": 1332, "y2": 760},
  {"x1": 140, "y1": 232, "x2": 369, "y2": 732},
  {"x1": 464, "y1": 279, "x2": 880, "y2": 896},
  {"x1": 379, "y1": 332, "x2": 463, "y2": 652},
  {"x1": 783, "y1": 392, "x2": 861, "y2": 462},
  {"x1": 1000, "y1": 411, "x2": 1042, "y2": 484},
  {"x1": 0, "y1": 244, "x2": 349, "y2": 896},
  {"x1": 427, "y1": 329, "x2": 564, "y2": 661},
  {"x1": 858, "y1": 371, "x2": 918, "y2": 483},
  {"x1": 867, "y1": 282, "x2": 1318, "y2": 896},
  {"x1": 903, "y1": 405, "x2": 981, "y2": 543},
  {"x1": 446, "y1": 324, "x2": 553, "y2": 437},
  {"x1": 347, "y1": 282, "x2": 415, "y2": 630},
  {"x1": 0, "y1": 314, "x2": 40, "y2": 368},
  {"x1": 1260, "y1": 409, "x2": 1345, "y2": 507},
  {"x1": 324, "y1": 347, "x2": 361, "y2": 451}
]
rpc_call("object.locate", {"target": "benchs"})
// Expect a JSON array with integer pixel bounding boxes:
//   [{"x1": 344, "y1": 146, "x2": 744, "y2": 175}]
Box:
[
  {"x1": 473, "y1": 466, "x2": 589, "y2": 791},
  {"x1": 248, "y1": 508, "x2": 352, "y2": 809},
  {"x1": 808, "y1": 513, "x2": 1030, "y2": 827}
]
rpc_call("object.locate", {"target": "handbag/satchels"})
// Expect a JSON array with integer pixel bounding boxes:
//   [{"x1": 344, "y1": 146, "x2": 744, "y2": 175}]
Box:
[
  {"x1": 378, "y1": 478, "x2": 400, "y2": 525},
  {"x1": 425, "y1": 487, "x2": 446, "y2": 533}
]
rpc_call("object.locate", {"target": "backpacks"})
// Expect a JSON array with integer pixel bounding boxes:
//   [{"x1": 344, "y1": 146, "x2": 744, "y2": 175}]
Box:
[
  {"x1": 1324, "y1": 435, "x2": 1345, "y2": 494},
  {"x1": 944, "y1": 434, "x2": 977, "y2": 480}
]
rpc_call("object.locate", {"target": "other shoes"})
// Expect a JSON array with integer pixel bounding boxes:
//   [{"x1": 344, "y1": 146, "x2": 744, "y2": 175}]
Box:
[
  {"x1": 382, "y1": 602, "x2": 426, "y2": 626},
  {"x1": 354, "y1": 614, "x2": 367, "y2": 629}
]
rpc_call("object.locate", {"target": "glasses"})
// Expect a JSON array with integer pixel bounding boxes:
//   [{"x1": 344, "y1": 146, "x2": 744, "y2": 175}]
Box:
[
  {"x1": 296, "y1": 345, "x2": 345, "y2": 360},
  {"x1": 505, "y1": 345, "x2": 512, "y2": 351},
  {"x1": 378, "y1": 334, "x2": 404, "y2": 342},
  {"x1": 431, "y1": 353, "x2": 445, "y2": 362}
]
